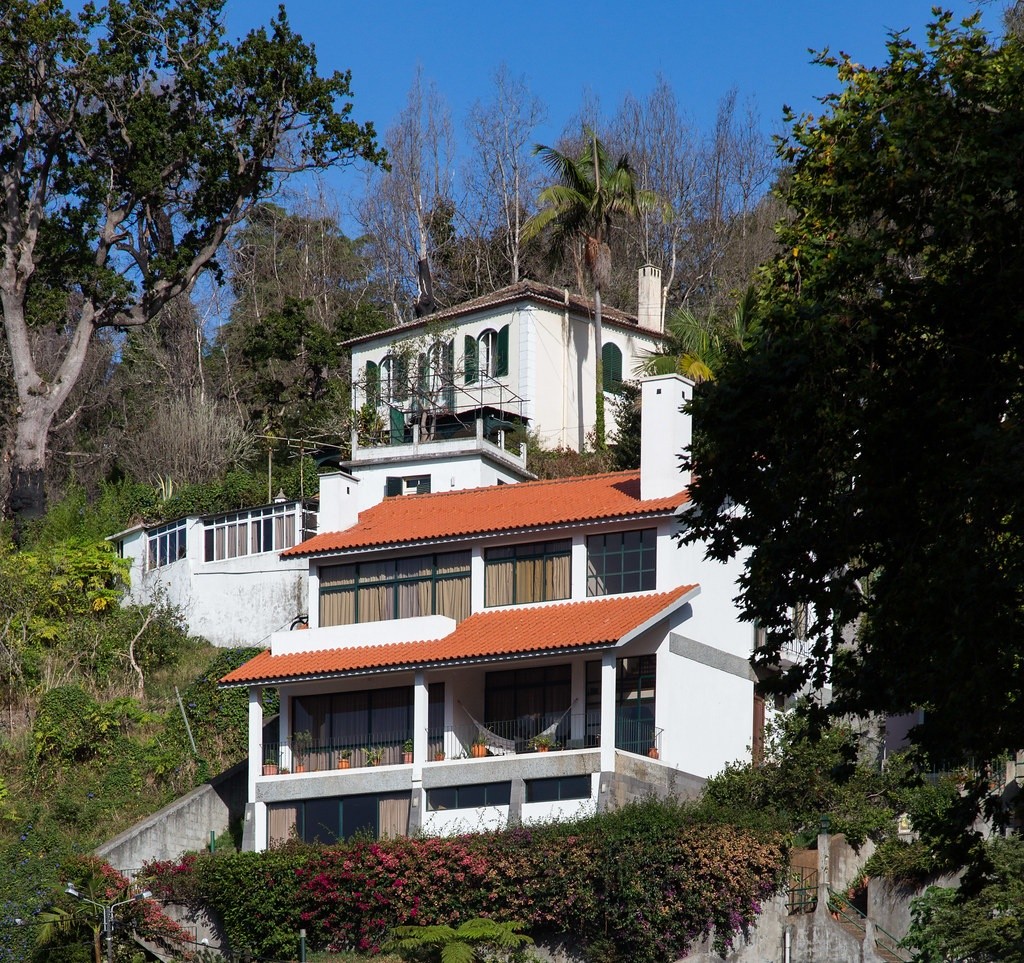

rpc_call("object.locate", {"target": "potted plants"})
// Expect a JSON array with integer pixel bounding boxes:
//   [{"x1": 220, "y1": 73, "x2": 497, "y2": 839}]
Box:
[
  {"x1": 338, "y1": 748, "x2": 352, "y2": 769},
  {"x1": 527, "y1": 733, "x2": 562, "y2": 752},
  {"x1": 287, "y1": 729, "x2": 313, "y2": 773},
  {"x1": 434, "y1": 751, "x2": 446, "y2": 761},
  {"x1": 470, "y1": 732, "x2": 491, "y2": 758},
  {"x1": 401, "y1": 738, "x2": 413, "y2": 764},
  {"x1": 279, "y1": 767, "x2": 290, "y2": 774},
  {"x1": 360, "y1": 745, "x2": 386, "y2": 767},
  {"x1": 259, "y1": 744, "x2": 283, "y2": 775}
]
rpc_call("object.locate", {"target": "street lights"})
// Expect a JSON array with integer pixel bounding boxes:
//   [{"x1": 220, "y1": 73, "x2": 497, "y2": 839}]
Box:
[{"x1": 65, "y1": 888, "x2": 152, "y2": 963}]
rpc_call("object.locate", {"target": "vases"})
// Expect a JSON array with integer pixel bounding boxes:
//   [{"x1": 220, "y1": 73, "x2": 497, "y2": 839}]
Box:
[{"x1": 648, "y1": 746, "x2": 659, "y2": 759}]
